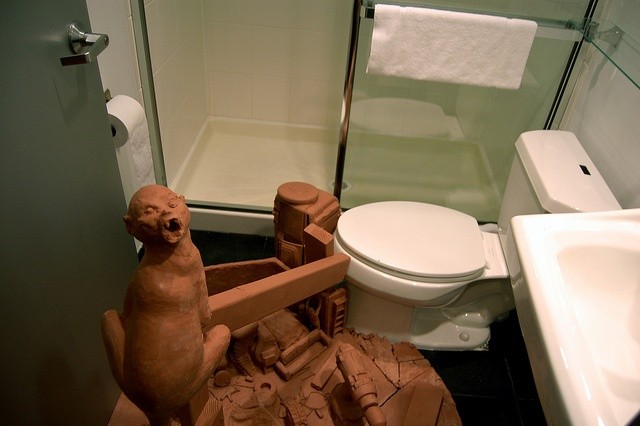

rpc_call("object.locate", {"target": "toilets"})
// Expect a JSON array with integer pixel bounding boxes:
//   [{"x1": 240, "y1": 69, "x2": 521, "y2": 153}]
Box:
[
  {"x1": 334, "y1": 130, "x2": 624, "y2": 351},
  {"x1": 349, "y1": 65, "x2": 542, "y2": 190}
]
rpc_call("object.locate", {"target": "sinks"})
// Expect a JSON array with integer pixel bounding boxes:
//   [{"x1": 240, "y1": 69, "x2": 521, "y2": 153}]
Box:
[{"x1": 508, "y1": 208, "x2": 640, "y2": 425}]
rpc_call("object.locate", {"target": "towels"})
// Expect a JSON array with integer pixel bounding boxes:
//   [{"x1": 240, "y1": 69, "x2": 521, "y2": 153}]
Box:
[{"x1": 364, "y1": 3, "x2": 538, "y2": 90}]
